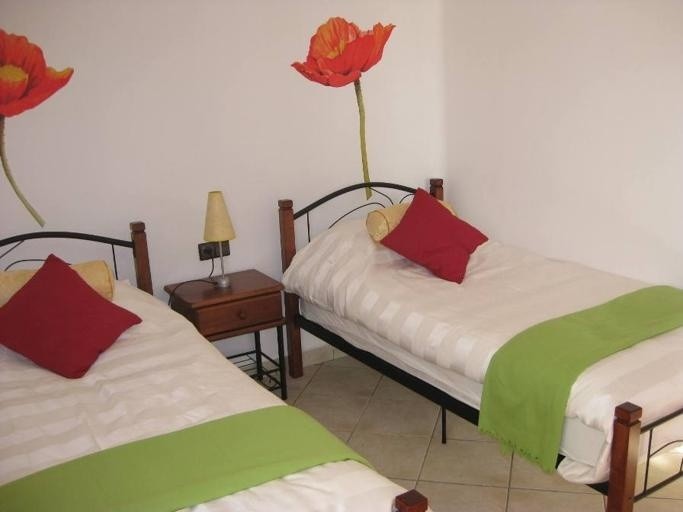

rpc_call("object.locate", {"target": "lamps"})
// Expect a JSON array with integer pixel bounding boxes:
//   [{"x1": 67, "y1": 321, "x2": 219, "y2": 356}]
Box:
[{"x1": 203, "y1": 190, "x2": 236, "y2": 287}]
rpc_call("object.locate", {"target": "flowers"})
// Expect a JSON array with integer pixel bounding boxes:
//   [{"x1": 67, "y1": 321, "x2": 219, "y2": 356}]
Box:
[
  {"x1": 291, "y1": 16, "x2": 397, "y2": 200},
  {"x1": 1, "y1": 27, "x2": 73, "y2": 228}
]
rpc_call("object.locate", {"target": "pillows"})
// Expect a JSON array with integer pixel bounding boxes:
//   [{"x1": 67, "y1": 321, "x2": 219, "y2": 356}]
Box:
[
  {"x1": 379, "y1": 187, "x2": 488, "y2": 284},
  {"x1": 1, "y1": 259, "x2": 114, "y2": 306},
  {"x1": 1, "y1": 253, "x2": 142, "y2": 379},
  {"x1": 365, "y1": 200, "x2": 455, "y2": 242}
]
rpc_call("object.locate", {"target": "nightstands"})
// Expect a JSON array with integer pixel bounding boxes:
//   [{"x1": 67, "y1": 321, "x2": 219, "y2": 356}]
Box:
[{"x1": 163, "y1": 270, "x2": 286, "y2": 402}]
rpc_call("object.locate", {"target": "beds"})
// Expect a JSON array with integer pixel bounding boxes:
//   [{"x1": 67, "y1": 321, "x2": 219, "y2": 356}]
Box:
[
  {"x1": 278, "y1": 178, "x2": 683, "y2": 512},
  {"x1": 0, "y1": 221, "x2": 429, "y2": 512}
]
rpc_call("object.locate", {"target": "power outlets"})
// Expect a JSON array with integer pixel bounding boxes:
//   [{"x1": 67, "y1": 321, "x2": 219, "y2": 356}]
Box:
[{"x1": 198, "y1": 242, "x2": 230, "y2": 262}]
